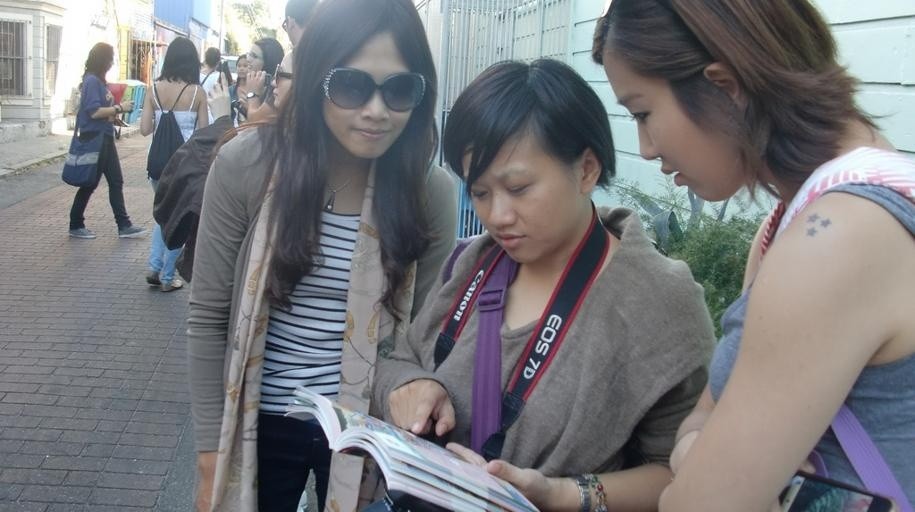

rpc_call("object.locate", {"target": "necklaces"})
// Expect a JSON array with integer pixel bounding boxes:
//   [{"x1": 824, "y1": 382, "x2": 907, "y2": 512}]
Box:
[{"x1": 319, "y1": 167, "x2": 353, "y2": 214}]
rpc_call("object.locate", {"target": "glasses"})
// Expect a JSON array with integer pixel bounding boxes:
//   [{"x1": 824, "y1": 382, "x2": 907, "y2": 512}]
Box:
[
  {"x1": 322, "y1": 67, "x2": 427, "y2": 113},
  {"x1": 282, "y1": 20, "x2": 288, "y2": 32},
  {"x1": 274, "y1": 63, "x2": 293, "y2": 84},
  {"x1": 246, "y1": 52, "x2": 260, "y2": 59}
]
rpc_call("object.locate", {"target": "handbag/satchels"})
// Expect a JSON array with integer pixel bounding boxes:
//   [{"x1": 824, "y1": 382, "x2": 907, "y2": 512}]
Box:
[{"x1": 62, "y1": 129, "x2": 105, "y2": 187}]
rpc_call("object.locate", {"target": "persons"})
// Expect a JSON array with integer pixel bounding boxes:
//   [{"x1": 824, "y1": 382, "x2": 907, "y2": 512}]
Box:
[
  {"x1": 184, "y1": 0, "x2": 461, "y2": 511},
  {"x1": 70, "y1": 42, "x2": 147, "y2": 239},
  {"x1": 141, "y1": 35, "x2": 326, "y2": 295},
  {"x1": 586, "y1": 2, "x2": 915, "y2": 512},
  {"x1": 373, "y1": 58, "x2": 716, "y2": 509}
]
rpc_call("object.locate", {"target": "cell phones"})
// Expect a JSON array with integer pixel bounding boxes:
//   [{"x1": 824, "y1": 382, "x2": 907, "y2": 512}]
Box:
[
  {"x1": 265, "y1": 73, "x2": 271, "y2": 85},
  {"x1": 779, "y1": 471, "x2": 897, "y2": 512}
]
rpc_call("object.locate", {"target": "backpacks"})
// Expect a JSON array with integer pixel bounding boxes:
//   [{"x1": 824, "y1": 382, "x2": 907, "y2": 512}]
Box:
[{"x1": 146, "y1": 84, "x2": 199, "y2": 181}]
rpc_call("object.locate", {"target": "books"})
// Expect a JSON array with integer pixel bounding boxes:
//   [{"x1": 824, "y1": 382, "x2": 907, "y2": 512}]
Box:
[{"x1": 283, "y1": 384, "x2": 540, "y2": 510}]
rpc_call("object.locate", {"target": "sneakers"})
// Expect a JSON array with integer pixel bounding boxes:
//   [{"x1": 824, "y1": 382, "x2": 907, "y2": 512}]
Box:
[
  {"x1": 118, "y1": 225, "x2": 147, "y2": 238},
  {"x1": 146, "y1": 276, "x2": 162, "y2": 284},
  {"x1": 70, "y1": 228, "x2": 97, "y2": 239},
  {"x1": 161, "y1": 279, "x2": 183, "y2": 292}
]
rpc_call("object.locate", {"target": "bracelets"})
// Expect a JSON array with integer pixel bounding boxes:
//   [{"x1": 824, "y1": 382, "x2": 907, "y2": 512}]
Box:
[{"x1": 571, "y1": 471, "x2": 608, "y2": 512}]
